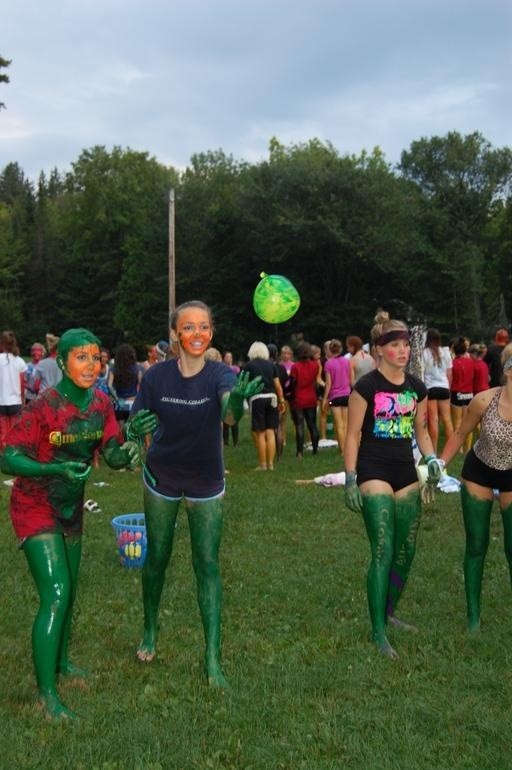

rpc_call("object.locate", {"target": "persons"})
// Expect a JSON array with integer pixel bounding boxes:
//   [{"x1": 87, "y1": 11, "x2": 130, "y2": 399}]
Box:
[
  {"x1": 280, "y1": 346, "x2": 296, "y2": 447},
  {"x1": 125, "y1": 302, "x2": 265, "y2": 690},
  {"x1": 345, "y1": 337, "x2": 376, "y2": 389},
  {"x1": 107, "y1": 344, "x2": 144, "y2": 440},
  {"x1": 311, "y1": 345, "x2": 326, "y2": 390},
  {"x1": 142, "y1": 347, "x2": 159, "y2": 450},
  {"x1": 344, "y1": 309, "x2": 441, "y2": 665},
  {"x1": 266, "y1": 344, "x2": 286, "y2": 455},
  {"x1": 203, "y1": 348, "x2": 221, "y2": 363},
  {"x1": 23, "y1": 343, "x2": 46, "y2": 402},
  {"x1": 450, "y1": 338, "x2": 474, "y2": 451},
  {"x1": 288, "y1": 341, "x2": 320, "y2": 459},
  {"x1": 28, "y1": 340, "x2": 63, "y2": 400},
  {"x1": 93, "y1": 347, "x2": 110, "y2": 396},
  {"x1": 468, "y1": 344, "x2": 490, "y2": 430},
  {"x1": 223, "y1": 352, "x2": 238, "y2": 446},
  {"x1": 0, "y1": 331, "x2": 30, "y2": 417},
  {"x1": 420, "y1": 343, "x2": 511, "y2": 635},
  {"x1": 483, "y1": 328, "x2": 508, "y2": 387},
  {"x1": 0, "y1": 330, "x2": 140, "y2": 720},
  {"x1": 239, "y1": 341, "x2": 278, "y2": 471},
  {"x1": 422, "y1": 329, "x2": 453, "y2": 455},
  {"x1": 323, "y1": 340, "x2": 350, "y2": 457}
]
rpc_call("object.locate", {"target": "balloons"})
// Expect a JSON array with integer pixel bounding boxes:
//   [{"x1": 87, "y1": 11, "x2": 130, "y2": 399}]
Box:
[{"x1": 253, "y1": 272, "x2": 300, "y2": 324}]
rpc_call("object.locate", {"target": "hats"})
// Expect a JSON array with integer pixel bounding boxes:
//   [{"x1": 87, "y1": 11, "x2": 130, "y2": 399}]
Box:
[{"x1": 496, "y1": 328, "x2": 510, "y2": 344}]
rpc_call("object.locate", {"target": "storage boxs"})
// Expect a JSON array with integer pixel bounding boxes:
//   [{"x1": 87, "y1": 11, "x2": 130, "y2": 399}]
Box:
[{"x1": 111, "y1": 513, "x2": 178, "y2": 573}]
